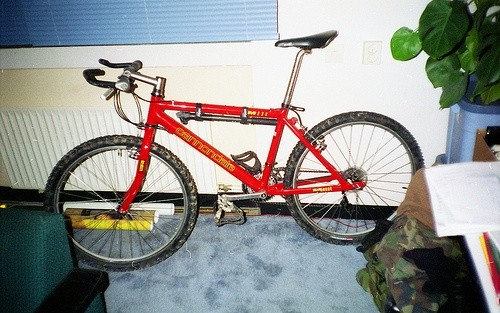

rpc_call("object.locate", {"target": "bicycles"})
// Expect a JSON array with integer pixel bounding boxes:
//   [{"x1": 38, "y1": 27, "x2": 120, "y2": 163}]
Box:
[{"x1": 41, "y1": 29, "x2": 425, "y2": 273}]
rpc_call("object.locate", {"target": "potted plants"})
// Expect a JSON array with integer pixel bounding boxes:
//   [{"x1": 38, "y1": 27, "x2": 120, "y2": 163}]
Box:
[{"x1": 389, "y1": 0, "x2": 500, "y2": 163}]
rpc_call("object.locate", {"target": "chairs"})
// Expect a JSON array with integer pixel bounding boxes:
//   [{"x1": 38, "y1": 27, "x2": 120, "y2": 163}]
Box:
[{"x1": 0, "y1": 210, "x2": 110, "y2": 313}]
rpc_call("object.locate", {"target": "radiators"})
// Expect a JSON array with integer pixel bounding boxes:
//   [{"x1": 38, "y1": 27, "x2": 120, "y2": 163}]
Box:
[{"x1": 0, "y1": 108, "x2": 219, "y2": 198}]
